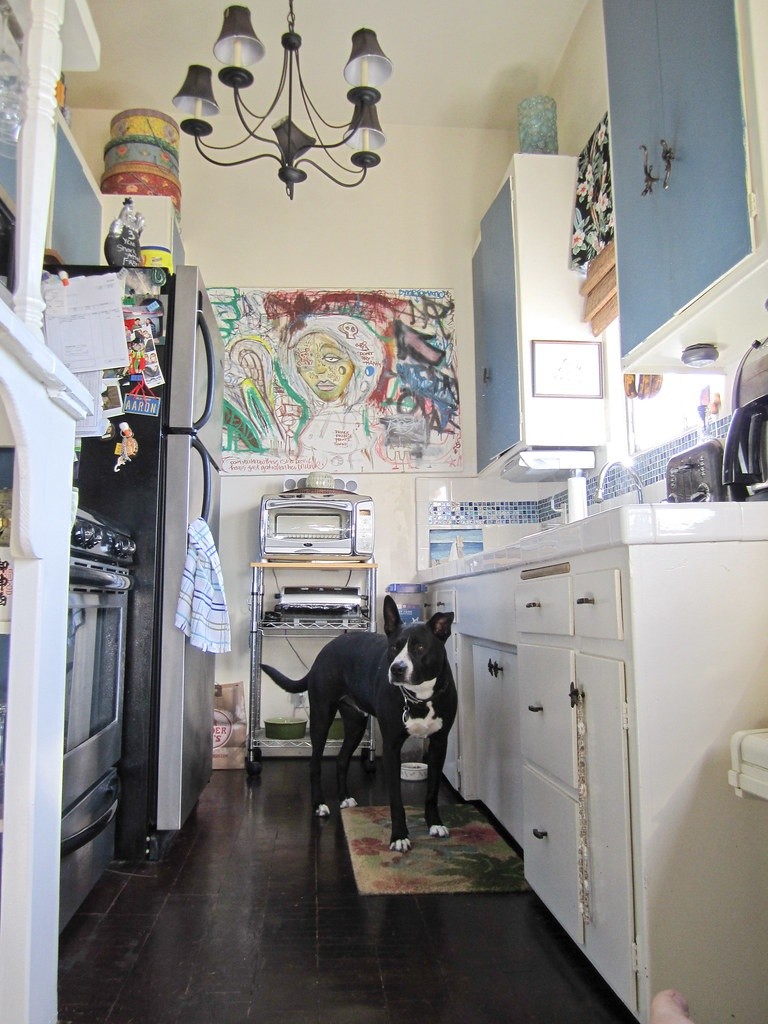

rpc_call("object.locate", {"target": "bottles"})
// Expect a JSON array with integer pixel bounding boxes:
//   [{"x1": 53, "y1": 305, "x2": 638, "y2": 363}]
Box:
[{"x1": 568, "y1": 468, "x2": 587, "y2": 524}]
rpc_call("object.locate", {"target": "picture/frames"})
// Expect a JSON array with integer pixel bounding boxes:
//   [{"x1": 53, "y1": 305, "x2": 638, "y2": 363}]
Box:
[{"x1": 531, "y1": 340, "x2": 603, "y2": 399}]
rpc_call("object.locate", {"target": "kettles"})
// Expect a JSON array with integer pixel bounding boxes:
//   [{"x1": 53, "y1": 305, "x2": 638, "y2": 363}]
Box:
[{"x1": 720, "y1": 394, "x2": 768, "y2": 502}]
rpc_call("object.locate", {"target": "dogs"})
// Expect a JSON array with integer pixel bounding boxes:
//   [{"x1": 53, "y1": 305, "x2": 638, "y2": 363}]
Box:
[{"x1": 257, "y1": 595, "x2": 459, "y2": 853}]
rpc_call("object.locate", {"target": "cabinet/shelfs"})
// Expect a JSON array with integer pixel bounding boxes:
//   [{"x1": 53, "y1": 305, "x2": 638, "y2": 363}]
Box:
[
  {"x1": 0, "y1": 20, "x2": 187, "y2": 272},
  {"x1": 245, "y1": 563, "x2": 380, "y2": 774},
  {"x1": 470, "y1": 154, "x2": 608, "y2": 480},
  {"x1": 604, "y1": 1, "x2": 768, "y2": 376},
  {"x1": 415, "y1": 543, "x2": 768, "y2": 1024},
  {"x1": 0, "y1": 0, "x2": 93, "y2": 1024}
]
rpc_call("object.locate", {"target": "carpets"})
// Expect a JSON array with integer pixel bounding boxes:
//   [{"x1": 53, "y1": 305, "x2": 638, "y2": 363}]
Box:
[{"x1": 340, "y1": 804, "x2": 528, "y2": 895}]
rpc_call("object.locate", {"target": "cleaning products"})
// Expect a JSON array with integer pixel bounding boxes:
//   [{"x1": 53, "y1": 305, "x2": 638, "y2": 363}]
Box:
[{"x1": 568, "y1": 468, "x2": 588, "y2": 524}]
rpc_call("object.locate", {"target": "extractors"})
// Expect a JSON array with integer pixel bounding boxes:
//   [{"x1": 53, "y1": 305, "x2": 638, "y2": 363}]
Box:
[{"x1": 497, "y1": 450, "x2": 596, "y2": 483}]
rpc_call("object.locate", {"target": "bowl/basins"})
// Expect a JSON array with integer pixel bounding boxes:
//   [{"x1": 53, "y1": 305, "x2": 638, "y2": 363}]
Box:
[
  {"x1": 306, "y1": 471, "x2": 335, "y2": 489},
  {"x1": 328, "y1": 718, "x2": 345, "y2": 739},
  {"x1": 400, "y1": 762, "x2": 431, "y2": 780},
  {"x1": 263, "y1": 717, "x2": 308, "y2": 739}
]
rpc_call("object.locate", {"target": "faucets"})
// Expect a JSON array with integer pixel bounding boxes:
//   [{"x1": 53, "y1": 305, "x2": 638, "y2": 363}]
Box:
[{"x1": 595, "y1": 460, "x2": 643, "y2": 504}]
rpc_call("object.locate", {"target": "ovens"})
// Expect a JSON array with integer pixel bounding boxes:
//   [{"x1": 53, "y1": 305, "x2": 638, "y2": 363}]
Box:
[
  {"x1": 58, "y1": 559, "x2": 134, "y2": 928},
  {"x1": 259, "y1": 488, "x2": 374, "y2": 560}
]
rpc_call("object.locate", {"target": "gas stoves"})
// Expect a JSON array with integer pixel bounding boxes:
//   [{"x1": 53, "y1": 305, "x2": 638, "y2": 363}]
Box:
[{"x1": 70, "y1": 504, "x2": 136, "y2": 565}]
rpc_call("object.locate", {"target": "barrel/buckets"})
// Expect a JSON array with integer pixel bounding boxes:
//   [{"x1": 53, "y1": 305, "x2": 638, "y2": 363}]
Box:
[{"x1": 385, "y1": 584, "x2": 427, "y2": 624}]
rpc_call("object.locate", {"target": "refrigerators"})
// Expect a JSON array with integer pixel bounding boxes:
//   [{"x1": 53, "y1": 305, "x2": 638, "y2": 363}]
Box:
[{"x1": 41, "y1": 264, "x2": 223, "y2": 862}]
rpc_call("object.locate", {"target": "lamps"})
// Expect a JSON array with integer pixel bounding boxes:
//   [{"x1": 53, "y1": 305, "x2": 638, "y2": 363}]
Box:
[{"x1": 172, "y1": 1, "x2": 394, "y2": 201}]
